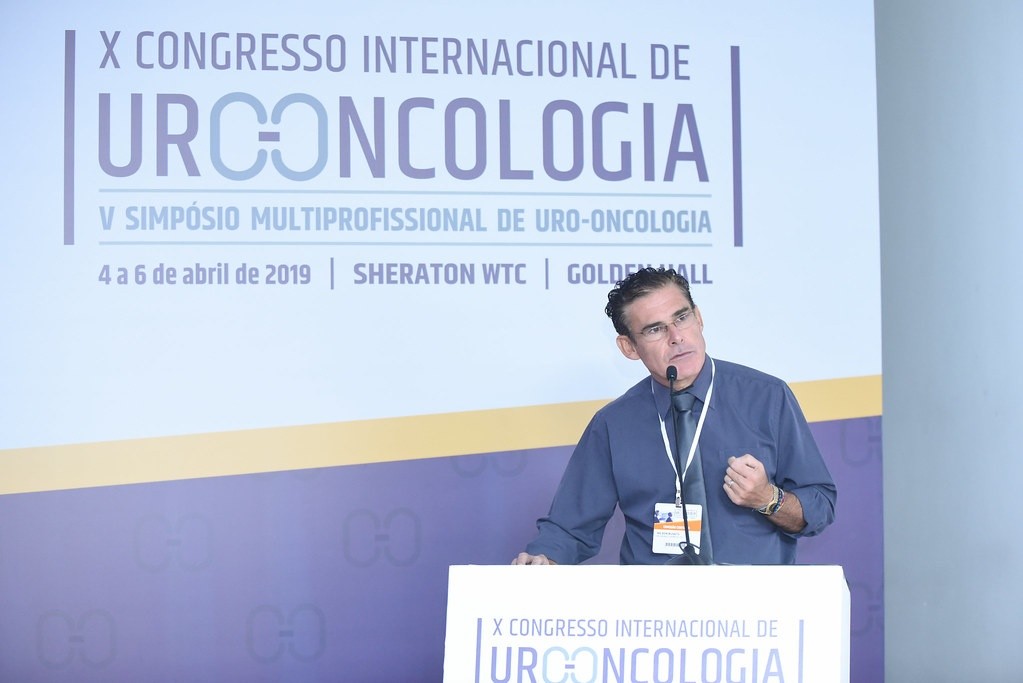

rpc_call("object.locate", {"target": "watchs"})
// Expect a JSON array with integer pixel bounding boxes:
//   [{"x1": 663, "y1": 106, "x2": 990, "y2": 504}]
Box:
[{"x1": 757, "y1": 484, "x2": 779, "y2": 516}]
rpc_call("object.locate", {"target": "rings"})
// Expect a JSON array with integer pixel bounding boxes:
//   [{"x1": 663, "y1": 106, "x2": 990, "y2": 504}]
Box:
[{"x1": 728, "y1": 479, "x2": 734, "y2": 487}]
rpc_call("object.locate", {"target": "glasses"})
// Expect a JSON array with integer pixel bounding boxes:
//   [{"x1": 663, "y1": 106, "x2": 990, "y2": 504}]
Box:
[{"x1": 630, "y1": 311, "x2": 697, "y2": 343}]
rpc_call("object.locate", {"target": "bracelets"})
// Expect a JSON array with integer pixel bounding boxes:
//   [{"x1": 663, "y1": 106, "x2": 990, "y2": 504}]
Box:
[{"x1": 753, "y1": 487, "x2": 786, "y2": 515}]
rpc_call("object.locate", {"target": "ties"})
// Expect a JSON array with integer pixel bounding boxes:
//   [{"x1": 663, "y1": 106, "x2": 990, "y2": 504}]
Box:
[{"x1": 673, "y1": 393, "x2": 712, "y2": 564}]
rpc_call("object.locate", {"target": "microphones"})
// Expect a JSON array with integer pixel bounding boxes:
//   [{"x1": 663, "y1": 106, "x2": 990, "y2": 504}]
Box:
[{"x1": 665, "y1": 363, "x2": 717, "y2": 565}]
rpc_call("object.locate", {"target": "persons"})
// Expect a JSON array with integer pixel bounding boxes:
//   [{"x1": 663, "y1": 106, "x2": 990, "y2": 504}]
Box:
[
  {"x1": 511, "y1": 266, "x2": 839, "y2": 565},
  {"x1": 666, "y1": 512, "x2": 673, "y2": 522},
  {"x1": 654, "y1": 510, "x2": 660, "y2": 523}
]
